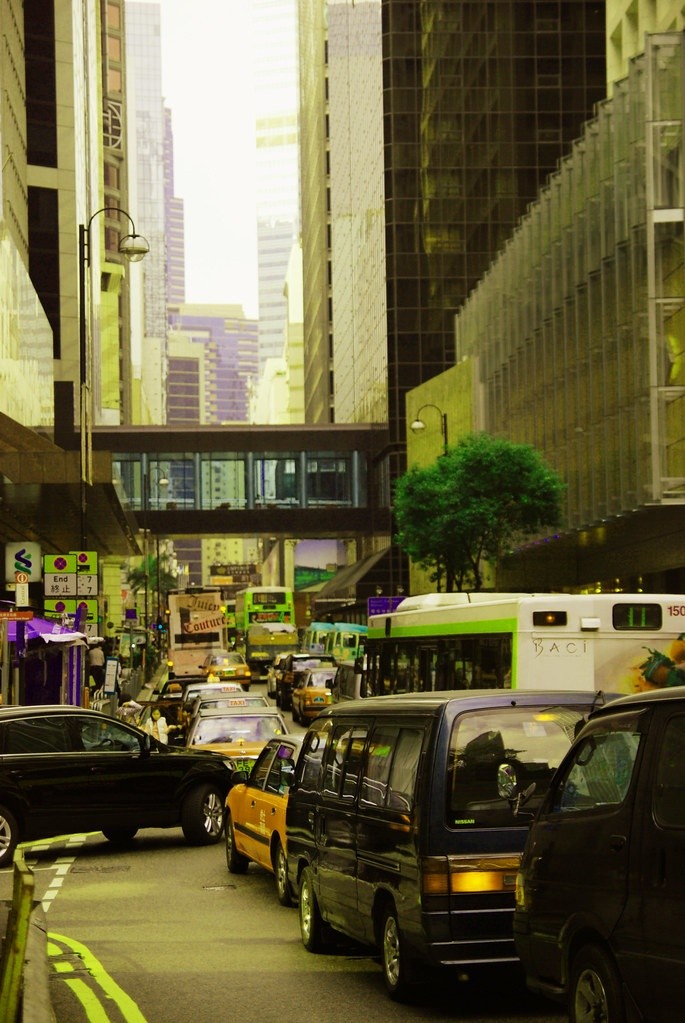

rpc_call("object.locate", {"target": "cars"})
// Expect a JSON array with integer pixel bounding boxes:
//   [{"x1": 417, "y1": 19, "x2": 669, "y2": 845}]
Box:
[
  {"x1": 223, "y1": 733, "x2": 308, "y2": 907},
  {"x1": 138, "y1": 648, "x2": 337, "y2": 766}
]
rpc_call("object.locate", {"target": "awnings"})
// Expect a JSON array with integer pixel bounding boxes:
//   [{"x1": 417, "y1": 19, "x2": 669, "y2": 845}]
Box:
[
  {"x1": 7, "y1": 619, "x2": 86, "y2": 643},
  {"x1": 311, "y1": 548, "x2": 398, "y2": 614}
]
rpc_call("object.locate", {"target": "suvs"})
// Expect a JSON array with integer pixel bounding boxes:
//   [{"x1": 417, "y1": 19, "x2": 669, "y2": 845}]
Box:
[{"x1": 0, "y1": 700, "x2": 240, "y2": 868}]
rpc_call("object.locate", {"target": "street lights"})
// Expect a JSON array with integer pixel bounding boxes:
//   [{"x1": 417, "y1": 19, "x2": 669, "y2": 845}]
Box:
[
  {"x1": 410, "y1": 404, "x2": 449, "y2": 456},
  {"x1": 80, "y1": 205, "x2": 149, "y2": 482}
]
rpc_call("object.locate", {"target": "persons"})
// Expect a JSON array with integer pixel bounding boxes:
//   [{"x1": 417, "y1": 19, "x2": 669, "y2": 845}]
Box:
[
  {"x1": 177, "y1": 705, "x2": 194, "y2": 731},
  {"x1": 114, "y1": 694, "x2": 141, "y2": 725},
  {"x1": 145, "y1": 708, "x2": 177, "y2": 745},
  {"x1": 89, "y1": 642, "x2": 105, "y2": 695}
]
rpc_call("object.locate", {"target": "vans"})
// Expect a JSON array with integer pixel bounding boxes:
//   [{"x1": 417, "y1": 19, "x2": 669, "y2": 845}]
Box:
[
  {"x1": 327, "y1": 657, "x2": 364, "y2": 703},
  {"x1": 284, "y1": 689, "x2": 645, "y2": 1010},
  {"x1": 495, "y1": 687, "x2": 685, "y2": 1023}
]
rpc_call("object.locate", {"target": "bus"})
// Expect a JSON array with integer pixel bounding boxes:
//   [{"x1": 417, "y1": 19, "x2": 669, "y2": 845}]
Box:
[
  {"x1": 227, "y1": 601, "x2": 239, "y2": 641},
  {"x1": 361, "y1": 593, "x2": 685, "y2": 693},
  {"x1": 302, "y1": 620, "x2": 368, "y2": 666},
  {"x1": 234, "y1": 585, "x2": 296, "y2": 636}
]
